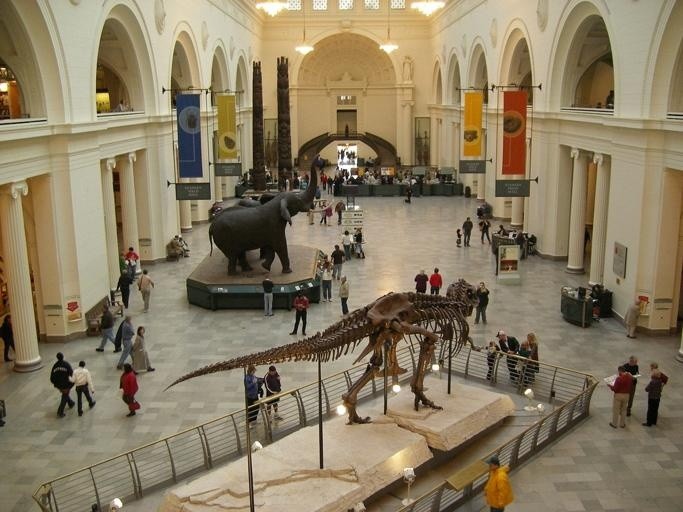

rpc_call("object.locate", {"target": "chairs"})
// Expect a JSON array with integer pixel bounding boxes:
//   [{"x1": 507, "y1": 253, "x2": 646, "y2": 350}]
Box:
[{"x1": 526, "y1": 233, "x2": 537, "y2": 255}]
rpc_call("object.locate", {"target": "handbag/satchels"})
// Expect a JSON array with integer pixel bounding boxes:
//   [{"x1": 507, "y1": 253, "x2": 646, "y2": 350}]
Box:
[{"x1": 129, "y1": 402, "x2": 140, "y2": 410}]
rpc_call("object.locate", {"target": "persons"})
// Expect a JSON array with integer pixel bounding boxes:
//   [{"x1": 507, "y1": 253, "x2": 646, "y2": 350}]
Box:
[
  {"x1": 124, "y1": 247, "x2": 139, "y2": 285},
  {"x1": 606, "y1": 90, "x2": 614, "y2": 109},
  {"x1": 68, "y1": 361, "x2": 97, "y2": 417},
  {"x1": 116, "y1": 269, "x2": 133, "y2": 309},
  {"x1": 623, "y1": 356, "x2": 639, "y2": 417},
  {"x1": 132, "y1": 327, "x2": 155, "y2": 372},
  {"x1": 484, "y1": 455, "x2": 514, "y2": 512},
  {"x1": 0, "y1": 314, "x2": 15, "y2": 361},
  {"x1": 96, "y1": 302, "x2": 122, "y2": 353},
  {"x1": 606, "y1": 366, "x2": 633, "y2": 429},
  {"x1": 642, "y1": 369, "x2": 661, "y2": 427},
  {"x1": 114, "y1": 99, "x2": 124, "y2": 112},
  {"x1": 137, "y1": 270, "x2": 155, "y2": 313},
  {"x1": 50, "y1": 352, "x2": 75, "y2": 417},
  {"x1": 650, "y1": 363, "x2": 669, "y2": 398},
  {"x1": 120, "y1": 364, "x2": 140, "y2": 417},
  {"x1": 116, "y1": 316, "x2": 135, "y2": 370},
  {"x1": 624, "y1": 299, "x2": 641, "y2": 339}
]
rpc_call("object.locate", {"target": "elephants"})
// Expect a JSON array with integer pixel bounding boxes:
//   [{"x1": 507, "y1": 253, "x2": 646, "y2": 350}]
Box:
[{"x1": 208, "y1": 154, "x2": 335, "y2": 276}]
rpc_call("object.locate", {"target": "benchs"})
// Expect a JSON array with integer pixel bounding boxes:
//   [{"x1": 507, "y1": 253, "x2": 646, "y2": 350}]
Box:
[
  {"x1": 165, "y1": 241, "x2": 183, "y2": 261},
  {"x1": 83, "y1": 296, "x2": 125, "y2": 334}
]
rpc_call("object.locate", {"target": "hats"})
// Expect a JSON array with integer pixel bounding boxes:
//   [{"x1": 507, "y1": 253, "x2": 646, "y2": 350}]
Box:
[{"x1": 486, "y1": 456, "x2": 499, "y2": 464}]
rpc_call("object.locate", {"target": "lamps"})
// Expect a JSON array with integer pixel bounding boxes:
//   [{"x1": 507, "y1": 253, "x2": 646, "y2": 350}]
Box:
[
  {"x1": 255, "y1": 0, "x2": 289, "y2": 20},
  {"x1": 410, "y1": 0, "x2": 444, "y2": 19},
  {"x1": 294, "y1": 1, "x2": 316, "y2": 57},
  {"x1": 250, "y1": 439, "x2": 262, "y2": 453},
  {"x1": 401, "y1": 466, "x2": 417, "y2": 506},
  {"x1": 377, "y1": 0, "x2": 400, "y2": 55},
  {"x1": 522, "y1": 388, "x2": 536, "y2": 411},
  {"x1": 536, "y1": 403, "x2": 544, "y2": 419},
  {"x1": 107, "y1": 497, "x2": 125, "y2": 512}
]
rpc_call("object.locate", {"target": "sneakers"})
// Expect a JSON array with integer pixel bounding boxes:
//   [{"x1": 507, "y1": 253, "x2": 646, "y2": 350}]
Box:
[{"x1": 78, "y1": 402, "x2": 96, "y2": 416}]
